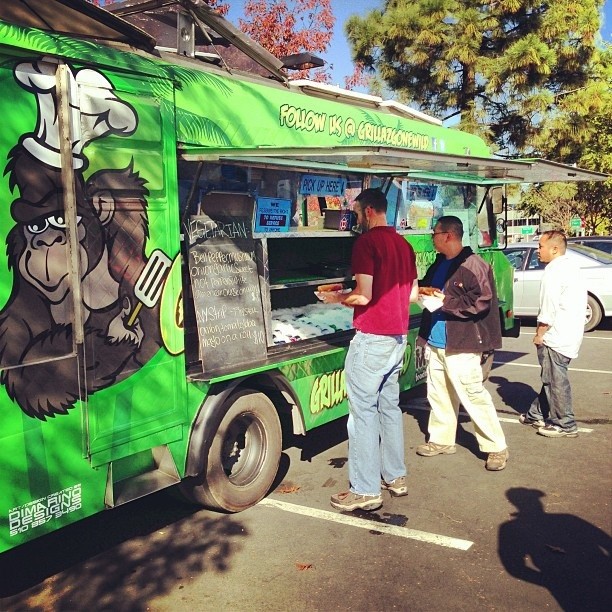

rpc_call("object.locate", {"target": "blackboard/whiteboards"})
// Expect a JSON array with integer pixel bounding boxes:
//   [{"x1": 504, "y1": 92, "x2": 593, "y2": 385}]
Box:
[{"x1": 183, "y1": 215, "x2": 267, "y2": 373}]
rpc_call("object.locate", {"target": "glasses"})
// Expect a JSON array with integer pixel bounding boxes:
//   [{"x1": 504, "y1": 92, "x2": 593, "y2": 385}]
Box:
[{"x1": 431, "y1": 233, "x2": 442, "y2": 238}]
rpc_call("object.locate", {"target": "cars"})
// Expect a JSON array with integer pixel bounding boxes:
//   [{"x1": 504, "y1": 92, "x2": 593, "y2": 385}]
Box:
[
  {"x1": 566, "y1": 237, "x2": 612, "y2": 253},
  {"x1": 496, "y1": 242, "x2": 612, "y2": 332}
]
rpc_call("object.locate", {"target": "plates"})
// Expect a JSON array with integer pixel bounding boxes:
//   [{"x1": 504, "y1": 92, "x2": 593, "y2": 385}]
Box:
[{"x1": 314, "y1": 289, "x2": 352, "y2": 301}]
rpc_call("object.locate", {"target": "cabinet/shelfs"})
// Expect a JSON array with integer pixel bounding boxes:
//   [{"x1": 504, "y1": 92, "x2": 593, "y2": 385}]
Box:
[{"x1": 247, "y1": 167, "x2": 371, "y2": 351}]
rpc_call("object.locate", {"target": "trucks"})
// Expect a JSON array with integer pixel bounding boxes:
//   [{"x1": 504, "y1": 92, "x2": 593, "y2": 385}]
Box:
[{"x1": 2, "y1": 1, "x2": 610, "y2": 553}]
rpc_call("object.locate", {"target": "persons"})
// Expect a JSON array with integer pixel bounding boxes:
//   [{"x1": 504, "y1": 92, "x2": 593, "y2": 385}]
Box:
[
  {"x1": 418, "y1": 215, "x2": 509, "y2": 471},
  {"x1": 519, "y1": 231, "x2": 588, "y2": 438},
  {"x1": 317, "y1": 188, "x2": 419, "y2": 512}
]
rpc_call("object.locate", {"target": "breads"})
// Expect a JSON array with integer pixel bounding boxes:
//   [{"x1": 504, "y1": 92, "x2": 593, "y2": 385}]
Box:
[
  {"x1": 417, "y1": 287, "x2": 440, "y2": 296},
  {"x1": 318, "y1": 284, "x2": 342, "y2": 292}
]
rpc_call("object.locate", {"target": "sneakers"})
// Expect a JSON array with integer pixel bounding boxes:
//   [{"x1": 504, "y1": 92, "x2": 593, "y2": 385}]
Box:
[
  {"x1": 538, "y1": 424, "x2": 578, "y2": 437},
  {"x1": 519, "y1": 414, "x2": 545, "y2": 429},
  {"x1": 330, "y1": 491, "x2": 383, "y2": 511},
  {"x1": 486, "y1": 449, "x2": 509, "y2": 470},
  {"x1": 416, "y1": 443, "x2": 456, "y2": 456},
  {"x1": 381, "y1": 477, "x2": 408, "y2": 497}
]
